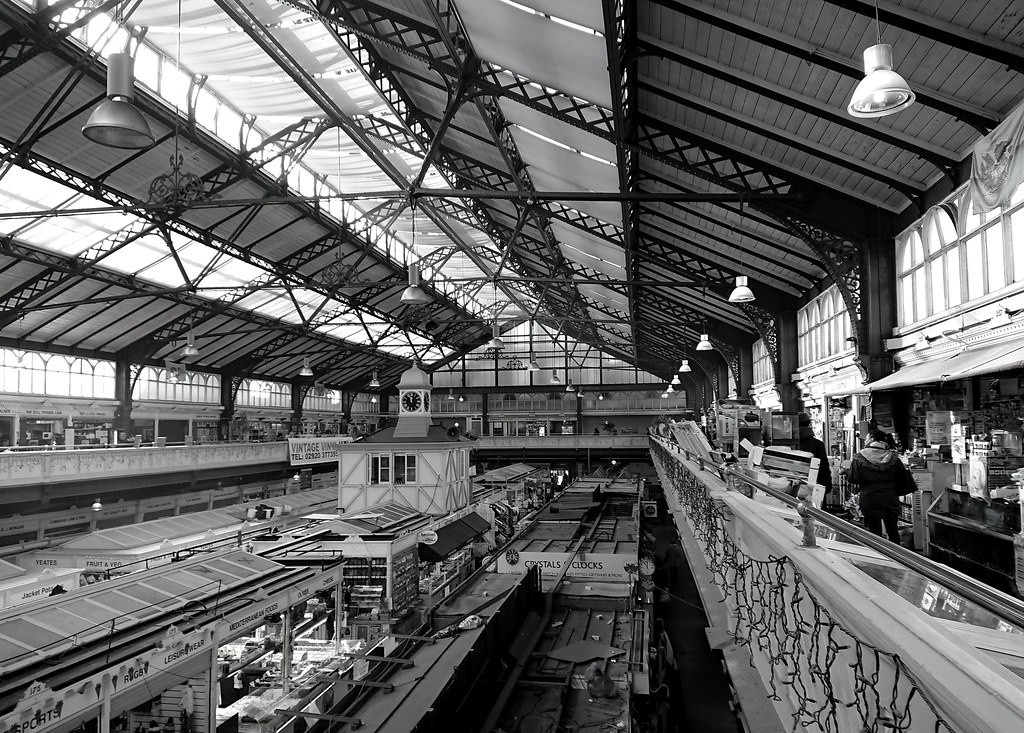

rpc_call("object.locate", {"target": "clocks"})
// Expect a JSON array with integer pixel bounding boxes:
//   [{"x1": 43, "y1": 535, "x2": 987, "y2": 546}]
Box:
[
  {"x1": 424, "y1": 392, "x2": 430, "y2": 412},
  {"x1": 482, "y1": 556, "x2": 496, "y2": 571},
  {"x1": 640, "y1": 559, "x2": 655, "y2": 575},
  {"x1": 401, "y1": 390, "x2": 423, "y2": 413}
]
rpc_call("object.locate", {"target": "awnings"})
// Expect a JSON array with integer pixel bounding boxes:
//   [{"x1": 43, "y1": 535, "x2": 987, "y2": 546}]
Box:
[
  {"x1": 419, "y1": 511, "x2": 492, "y2": 563},
  {"x1": 825, "y1": 337, "x2": 1024, "y2": 396}
]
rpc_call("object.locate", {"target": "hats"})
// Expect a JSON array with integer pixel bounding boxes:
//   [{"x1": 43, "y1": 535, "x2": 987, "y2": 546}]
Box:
[{"x1": 798, "y1": 413, "x2": 813, "y2": 423}]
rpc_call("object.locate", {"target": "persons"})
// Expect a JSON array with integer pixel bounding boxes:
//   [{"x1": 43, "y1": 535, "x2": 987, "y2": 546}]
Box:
[
  {"x1": 799, "y1": 413, "x2": 832, "y2": 511},
  {"x1": 593, "y1": 427, "x2": 600, "y2": 435},
  {"x1": 843, "y1": 430, "x2": 907, "y2": 545}
]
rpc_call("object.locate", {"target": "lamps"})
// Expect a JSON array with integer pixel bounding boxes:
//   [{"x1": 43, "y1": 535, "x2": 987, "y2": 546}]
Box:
[
  {"x1": 661, "y1": 390, "x2": 668, "y2": 398},
  {"x1": 667, "y1": 385, "x2": 676, "y2": 394},
  {"x1": 671, "y1": 375, "x2": 681, "y2": 385},
  {"x1": 370, "y1": 372, "x2": 380, "y2": 386},
  {"x1": 527, "y1": 353, "x2": 541, "y2": 372},
  {"x1": 847, "y1": 44, "x2": 916, "y2": 118},
  {"x1": 679, "y1": 359, "x2": 692, "y2": 372},
  {"x1": 577, "y1": 389, "x2": 584, "y2": 397},
  {"x1": 180, "y1": 333, "x2": 202, "y2": 356},
  {"x1": 81, "y1": 51, "x2": 154, "y2": 150},
  {"x1": 696, "y1": 334, "x2": 713, "y2": 350},
  {"x1": 299, "y1": 357, "x2": 314, "y2": 376},
  {"x1": 727, "y1": 275, "x2": 755, "y2": 302},
  {"x1": 488, "y1": 324, "x2": 505, "y2": 350},
  {"x1": 566, "y1": 378, "x2": 574, "y2": 392},
  {"x1": 550, "y1": 369, "x2": 561, "y2": 384},
  {"x1": 91, "y1": 502, "x2": 104, "y2": 511},
  {"x1": 399, "y1": 265, "x2": 428, "y2": 304}
]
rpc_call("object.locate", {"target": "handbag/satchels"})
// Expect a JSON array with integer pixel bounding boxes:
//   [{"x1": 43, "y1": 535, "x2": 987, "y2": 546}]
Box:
[{"x1": 898, "y1": 470, "x2": 919, "y2": 497}]
